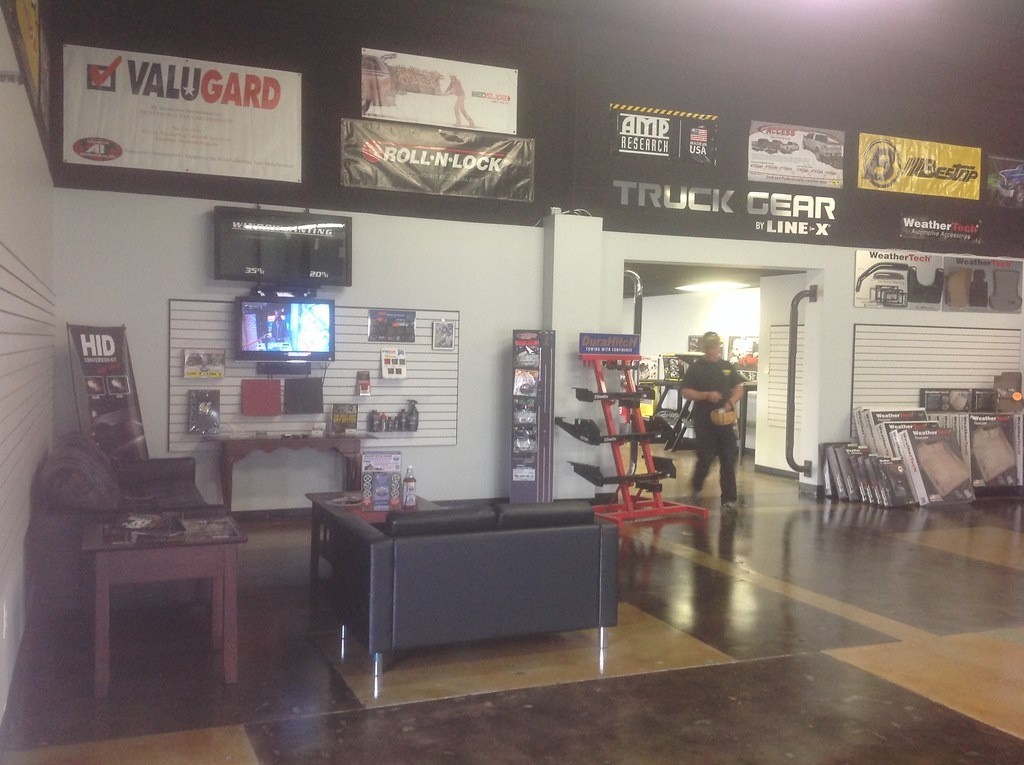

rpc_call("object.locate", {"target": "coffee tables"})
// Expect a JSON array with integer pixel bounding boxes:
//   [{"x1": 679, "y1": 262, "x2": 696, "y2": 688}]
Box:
[{"x1": 304, "y1": 490, "x2": 440, "y2": 596}]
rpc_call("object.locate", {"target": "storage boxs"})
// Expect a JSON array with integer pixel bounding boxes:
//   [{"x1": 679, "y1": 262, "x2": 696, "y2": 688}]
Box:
[
  {"x1": 639, "y1": 333, "x2": 760, "y2": 386},
  {"x1": 820, "y1": 371, "x2": 1024, "y2": 508},
  {"x1": 362, "y1": 472, "x2": 402, "y2": 511},
  {"x1": 359, "y1": 450, "x2": 402, "y2": 473}
]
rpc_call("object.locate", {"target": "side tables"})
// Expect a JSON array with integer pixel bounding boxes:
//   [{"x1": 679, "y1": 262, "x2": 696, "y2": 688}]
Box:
[{"x1": 79, "y1": 504, "x2": 248, "y2": 697}]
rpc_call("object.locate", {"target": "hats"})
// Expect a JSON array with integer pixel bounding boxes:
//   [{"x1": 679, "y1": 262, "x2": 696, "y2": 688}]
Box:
[{"x1": 702, "y1": 331, "x2": 721, "y2": 348}]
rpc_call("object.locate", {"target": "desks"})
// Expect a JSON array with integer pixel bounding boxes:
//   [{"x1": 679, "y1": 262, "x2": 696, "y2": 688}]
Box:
[
  {"x1": 654, "y1": 379, "x2": 758, "y2": 466},
  {"x1": 200, "y1": 433, "x2": 378, "y2": 518}
]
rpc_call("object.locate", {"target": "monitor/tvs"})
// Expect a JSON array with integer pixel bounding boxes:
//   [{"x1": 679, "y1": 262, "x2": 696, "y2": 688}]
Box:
[
  {"x1": 214, "y1": 206, "x2": 352, "y2": 287},
  {"x1": 235, "y1": 296, "x2": 335, "y2": 361}
]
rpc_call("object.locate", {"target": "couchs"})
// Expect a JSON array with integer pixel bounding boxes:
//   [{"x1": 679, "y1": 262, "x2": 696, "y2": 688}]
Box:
[
  {"x1": 34, "y1": 435, "x2": 227, "y2": 618},
  {"x1": 333, "y1": 500, "x2": 621, "y2": 689}
]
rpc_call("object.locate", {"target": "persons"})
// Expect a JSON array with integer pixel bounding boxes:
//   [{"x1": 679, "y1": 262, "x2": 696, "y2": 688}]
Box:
[
  {"x1": 679, "y1": 332, "x2": 749, "y2": 508},
  {"x1": 272, "y1": 310, "x2": 287, "y2": 342},
  {"x1": 437, "y1": 328, "x2": 452, "y2": 347},
  {"x1": 446, "y1": 73, "x2": 475, "y2": 128}
]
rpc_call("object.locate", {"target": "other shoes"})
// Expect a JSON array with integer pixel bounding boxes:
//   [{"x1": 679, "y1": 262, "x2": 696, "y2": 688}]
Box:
[
  {"x1": 721, "y1": 499, "x2": 737, "y2": 509},
  {"x1": 691, "y1": 483, "x2": 701, "y2": 501}
]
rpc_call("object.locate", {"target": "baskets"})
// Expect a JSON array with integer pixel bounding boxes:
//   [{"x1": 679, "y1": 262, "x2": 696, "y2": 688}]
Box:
[{"x1": 711, "y1": 398, "x2": 737, "y2": 425}]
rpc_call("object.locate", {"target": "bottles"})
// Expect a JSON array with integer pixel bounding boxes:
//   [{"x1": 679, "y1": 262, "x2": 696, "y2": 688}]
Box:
[
  {"x1": 369, "y1": 409, "x2": 408, "y2": 431},
  {"x1": 403, "y1": 465, "x2": 417, "y2": 511}
]
